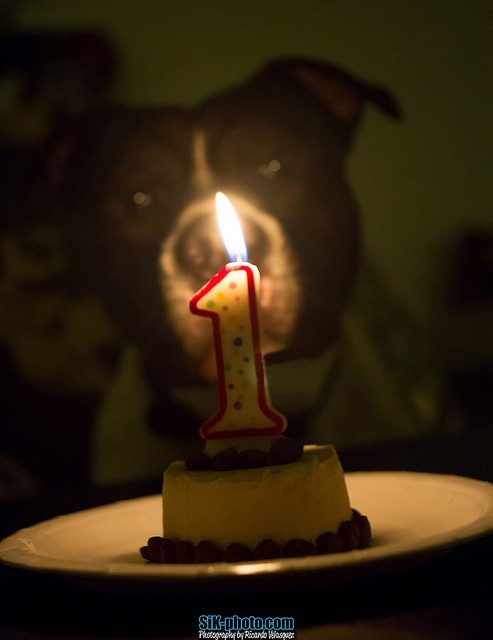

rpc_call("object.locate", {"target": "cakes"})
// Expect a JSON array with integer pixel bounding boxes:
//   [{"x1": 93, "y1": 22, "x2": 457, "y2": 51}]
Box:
[{"x1": 140, "y1": 442, "x2": 371, "y2": 558}]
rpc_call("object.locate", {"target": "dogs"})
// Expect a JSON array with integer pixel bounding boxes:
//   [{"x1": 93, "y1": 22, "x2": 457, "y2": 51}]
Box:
[{"x1": 36, "y1": 55, "x2": 443, "y2": 488}]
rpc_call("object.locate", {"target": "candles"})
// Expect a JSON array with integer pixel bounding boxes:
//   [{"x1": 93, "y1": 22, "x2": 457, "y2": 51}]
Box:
[{"x1": 188, "y1": 260, "x2": 288, "y2": 444}]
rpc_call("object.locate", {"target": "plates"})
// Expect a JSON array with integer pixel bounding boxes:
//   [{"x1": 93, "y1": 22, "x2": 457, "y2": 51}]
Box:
[{"x1": 0, "y1": 472, "x2": 492, "y2": 576}]
